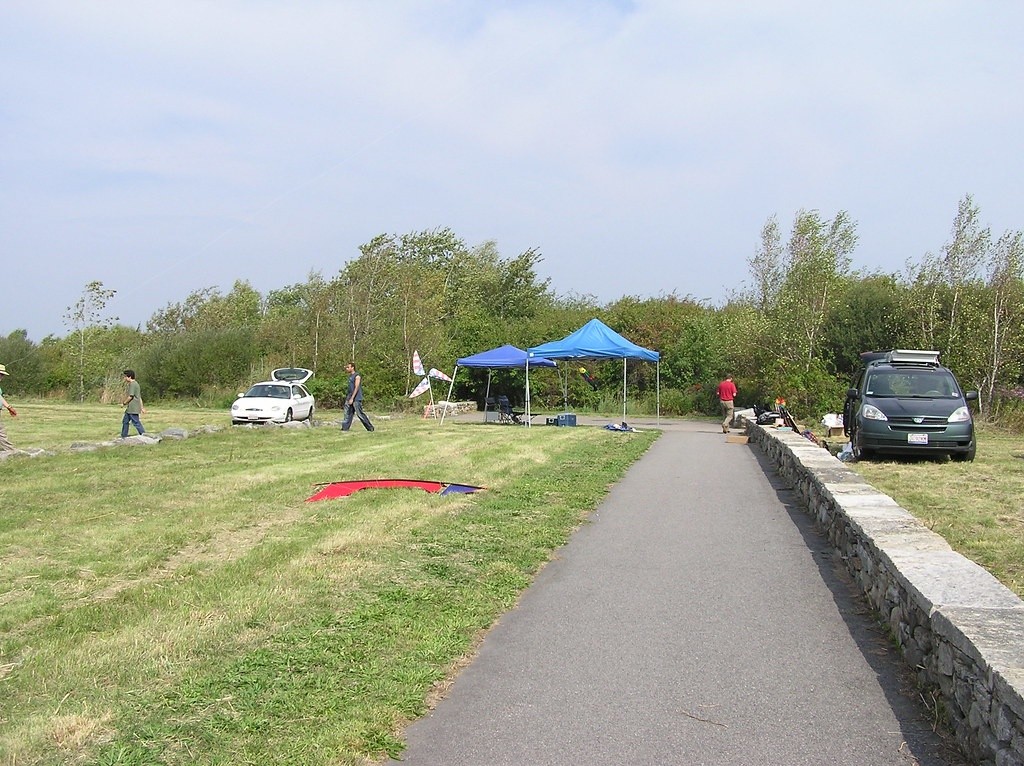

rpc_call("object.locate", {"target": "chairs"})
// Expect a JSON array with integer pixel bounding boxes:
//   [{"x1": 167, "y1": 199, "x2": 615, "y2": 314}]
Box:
[
  {"x1": 873, "y1": 378, "x2": 895, "y2": 394},
  {"x1": 915, "y1": 378, "x2": 936, "y2": 394},
  {"x1": 483, "y1": 395, "x2": 542, "y2": 426}
]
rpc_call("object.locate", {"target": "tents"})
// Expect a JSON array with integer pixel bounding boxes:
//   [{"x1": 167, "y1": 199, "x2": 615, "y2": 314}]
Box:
[
  {"x1": 440, "y1": 344, "x2": 565, "y2": 427},
  {"x1": 526, "y1": 318, "x2": 659, "y2": 430}
]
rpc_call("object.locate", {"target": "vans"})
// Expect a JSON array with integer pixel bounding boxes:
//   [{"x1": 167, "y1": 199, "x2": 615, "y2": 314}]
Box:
[{"x1": 842, "y1": 350, "x2": 979, "y2": 461}]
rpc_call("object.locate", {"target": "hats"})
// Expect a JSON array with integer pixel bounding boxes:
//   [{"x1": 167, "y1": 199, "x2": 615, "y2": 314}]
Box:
[{"x1": 0, "y1": 364, "x2": 9, "y2": 375}]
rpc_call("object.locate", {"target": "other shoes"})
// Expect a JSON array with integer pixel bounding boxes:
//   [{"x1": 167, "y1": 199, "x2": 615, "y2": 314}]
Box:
[
  {"x1": 722, "y1": 424, "x2": 727, "y2": 433},
  {"x1": 727, "y1": 431, "x2": 730, "y2": 433}
]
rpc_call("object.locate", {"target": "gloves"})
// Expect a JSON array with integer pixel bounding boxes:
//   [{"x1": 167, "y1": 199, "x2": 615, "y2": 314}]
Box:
[{"x1": 7, "y1": 405, "x2": 16, "y2": 417}]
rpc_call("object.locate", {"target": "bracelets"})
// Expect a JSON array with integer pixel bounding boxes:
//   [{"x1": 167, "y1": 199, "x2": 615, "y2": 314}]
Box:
[
  {"x1": 123, "y1": 402, "x2": 126, "y2": 405},
  {"x1": 8, "y1": 405, "x2": 11, "y2": 409}
]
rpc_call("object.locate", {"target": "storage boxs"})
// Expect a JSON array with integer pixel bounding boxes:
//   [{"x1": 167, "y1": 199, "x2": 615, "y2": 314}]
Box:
[
  {"x1": 557, "y1": 413, "x2": 576, "y2": 426},
  {"x1": 826, "y1": 426, "x2": 846, "y2": 438},
  {"x1": 546, "y1": 418, "x2": 558, "y2": 426}
]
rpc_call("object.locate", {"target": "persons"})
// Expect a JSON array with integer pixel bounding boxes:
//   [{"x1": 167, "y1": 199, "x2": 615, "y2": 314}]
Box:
[
  {"x1": 0, "y1": 364, "x2": 17, "y2": 451},
  {"x1": 119, "y1": 370, "x2": 145, "y2": 440},
  {"x1": 341, "y1": 363, "x2": 374, "y2": 431},
  {"x1": 717, "y1": 375, "x2": 736, "y2": 433}
]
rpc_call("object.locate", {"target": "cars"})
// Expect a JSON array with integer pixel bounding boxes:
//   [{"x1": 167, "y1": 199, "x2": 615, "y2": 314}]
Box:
[{"x1": 230, "y1": 368, "x2": 316, "y2": 425}]
